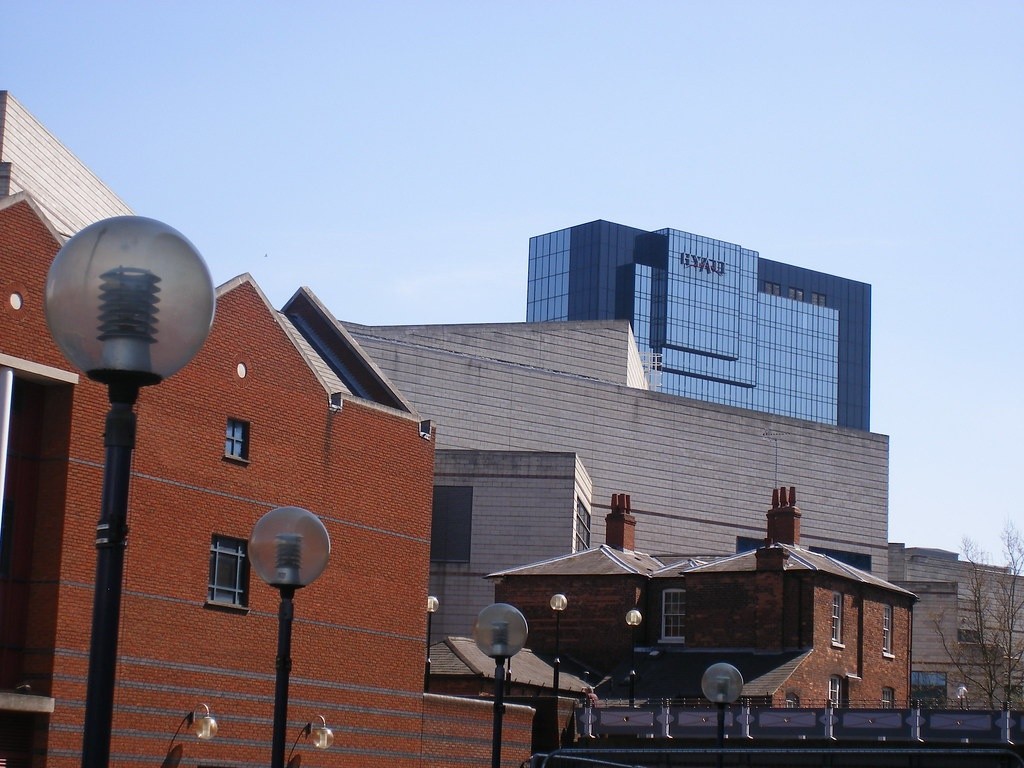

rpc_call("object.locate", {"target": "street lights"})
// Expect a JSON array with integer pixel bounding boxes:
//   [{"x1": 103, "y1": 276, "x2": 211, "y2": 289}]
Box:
[
  {"x1": 550, "y1": 592, "x2": 567, "y2": 720},
  {"x1": 474, "y1": 603, "x2": 529, "y2": 768},
  {"x1": 424, "y1": 595, "x2": 440, "y2": 694},
  {"x1": 702, "y1": 663, "x2": 743, "y2": 748},
  {"x1": 248, "y1": 507, "x2": 333, "y2": 767},
  {"x1": 43, "y1": 216, "x2": 217, "y2": 768},
  {"x1": 626, "y1": 609, "x2": 642, "y2": 708},
  {"x1": 955, "y1": 686, "x2": 968, "y2": 710}
]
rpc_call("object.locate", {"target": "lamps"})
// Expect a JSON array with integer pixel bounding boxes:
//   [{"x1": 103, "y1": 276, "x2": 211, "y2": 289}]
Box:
[
  {"x1": 307, "y1": 715, "x2": 335, "y2": 748},
  {"x1": 191, "y1": 704, "x2": 218, "y2": 739}
]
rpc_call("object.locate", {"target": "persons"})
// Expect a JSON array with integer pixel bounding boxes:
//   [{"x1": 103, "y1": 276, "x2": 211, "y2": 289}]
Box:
[{"x1": 585, "y1": 685, "x2": 598, "y2": 701}]
